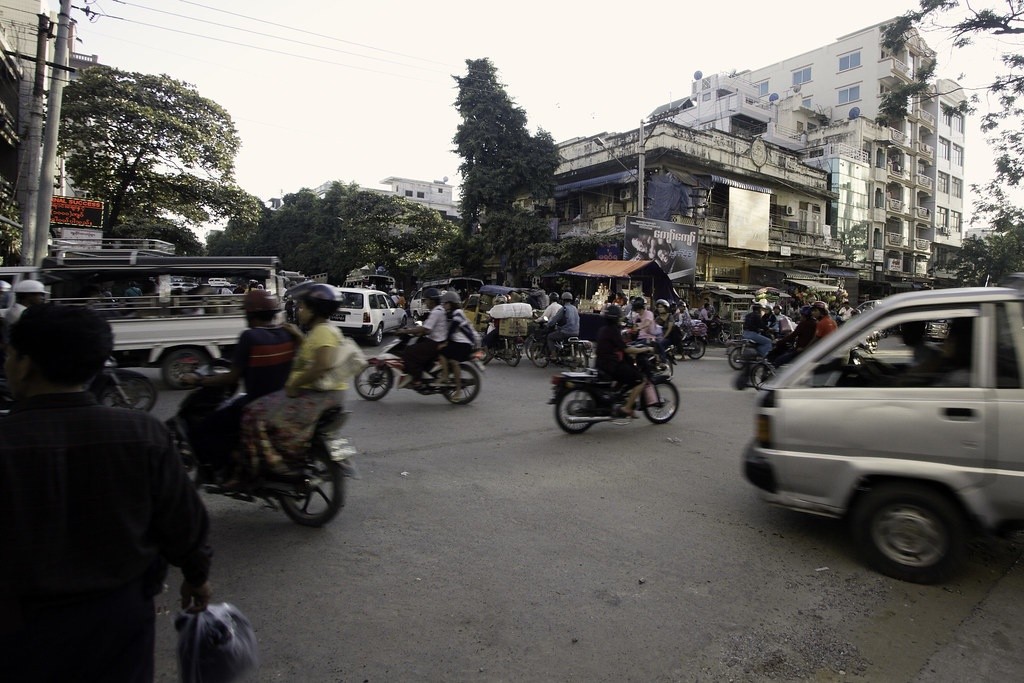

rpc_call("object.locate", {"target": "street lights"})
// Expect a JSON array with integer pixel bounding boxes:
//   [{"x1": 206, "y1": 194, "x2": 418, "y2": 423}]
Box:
[
  {"x1": 593, "y1": 137, "x2": 644, "y2": 217},
  {"x1": 868, "y1": 139, "x2": 895, "y2": 261}
]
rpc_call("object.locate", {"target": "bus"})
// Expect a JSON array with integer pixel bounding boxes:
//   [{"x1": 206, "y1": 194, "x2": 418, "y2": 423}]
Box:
[{"x1": 339, "y1": 274, "x2": 397, "y2": 297}]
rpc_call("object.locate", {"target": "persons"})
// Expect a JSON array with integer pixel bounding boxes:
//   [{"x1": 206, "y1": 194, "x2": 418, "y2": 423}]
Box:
[
  {"x1": 124, "y1": 281, "x2": 294, "y2": 324},
  {"x1": 177, "y1": 289, "x2": 303, "y2": 486},
  {"x1": 244, "y1": 284, "x2": 350, "y2": 476},
  {"x1": 773, "y1": 306, "x2": 792, "y2": 330},
  {"x1": 630, "y1": 231, "x2": 683, "y2": 273},
  {"x1": 796, "y1": 301, "x2": 853, "y2": 351},
  {"x1": 674, "y1": 299, "x2": 692, "y2": 361},
  {"x1": 626, "y1": 296, "x2": 638, "y2": 315},
  {"x1": 595, "y1": 306, "x2": 654, "y2": 419},
  {"x1": 698, "y1": 303, "x2": 713, "y2": 322},
  {"x1": 0, "y1": 278, "x2": 215, "y2": 683},
  {"x1": 867, "y1": 321, "x2": 968, "y2": 387},
  {"x1": 762, "y1": 306, "x2": 776, "y2": 328},
  {"x1": 774, "y1": 305, "x2": 816, "y2": 366},
  {"x1": 391, "y1": 287, "x2": 580, "y2": 402},
  {"x1": 655, "y1": 299, "x2": 679, "y2": 360},
  {"x1": 673, "y1": 301, "x2": 681, "y2": 322},
  {"x1": 699, "y1": 298, "x2": 716, "y2": 312},
  {"x1": 626, "y1": 301, "x2": 667, "y2": 368},
  {"x1": 742, "y1": 304, "x2": 773, "y2": 361}
]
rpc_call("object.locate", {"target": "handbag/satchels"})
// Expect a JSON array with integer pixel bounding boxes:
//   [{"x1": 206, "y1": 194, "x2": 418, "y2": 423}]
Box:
[
  {"x1": 556, "y1": 307, "x2": 567, "y2": 327},
  {"x1": 303, "y1": 326, "x2": 368, "y2": 392},
  {"x1": 174, "y1": 603, "x2": 260, "y2": 683}
]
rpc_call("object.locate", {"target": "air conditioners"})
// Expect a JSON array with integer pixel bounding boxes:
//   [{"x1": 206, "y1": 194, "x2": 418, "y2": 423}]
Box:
[
  {"x1": 941, "y1": 227, "x2": 947, "y2": 233},
  {"x1": 620, "y1": 187, "x2": 632, "y2": 200},
  {"x1": 783, "y1": 205, "x2": 795, "y2": 215}
]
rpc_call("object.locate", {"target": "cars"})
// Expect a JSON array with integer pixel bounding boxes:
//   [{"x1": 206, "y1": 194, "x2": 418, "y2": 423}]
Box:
[
  {"x1": 328, "y1": 287, "x2": 408, "y2": 345},
  {"x1": 856, "y1": 300, "x2": 880, "y2": 313}
]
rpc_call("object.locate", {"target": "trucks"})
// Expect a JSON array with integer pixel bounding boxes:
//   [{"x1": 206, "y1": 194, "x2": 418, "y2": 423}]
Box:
[
  {"x1": 461, "y1": 292, "x2": 546, "y2": 332},
  {"x1": 0, "y1": 238, "x2": 287, "y2": 390},
  {"x1": 741, "y1": 287, "x2": 1024, "y2": 580},
  {"x1": 409, "y1": 277, "x2": 484, "y2": 325}
]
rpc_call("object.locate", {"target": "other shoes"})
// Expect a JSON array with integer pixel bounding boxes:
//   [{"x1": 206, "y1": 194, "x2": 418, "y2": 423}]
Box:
[{"x1": 621, "y1": 408, "x2": 640, "y2": 419}]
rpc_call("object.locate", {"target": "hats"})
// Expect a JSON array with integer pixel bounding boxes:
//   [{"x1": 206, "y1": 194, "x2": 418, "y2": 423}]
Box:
[{"x1": 13, "y1": 278, "x2": 52, "y2": 294}]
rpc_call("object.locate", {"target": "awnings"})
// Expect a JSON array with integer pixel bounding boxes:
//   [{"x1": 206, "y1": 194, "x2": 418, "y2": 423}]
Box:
[{"x1": 558, "y1": 259, "x2": 656, "y2": 299}]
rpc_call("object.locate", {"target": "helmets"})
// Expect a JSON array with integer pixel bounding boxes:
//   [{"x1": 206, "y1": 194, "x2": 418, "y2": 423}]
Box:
[
  {"x1": 301, "y1": 283, "x2": 344, "y2": 302},
  {"x1": 604, "y1": 305, "x2": 624, "y2": 319},
  {"x1": 812, "y1": 300, "x2": 828, "y2": 309},
  {"x1": 421, "y1": 288, "x2": 441, "y2": 299},
  {"x1": 548, "y1": 292, "x2": 559, "y2": 298},
  {"x1": 656, "y1": 299, "x2": 670, "y2": 310},
  {"x1": 631, "y1": 296, "x2": 647, "y2": 305},
  {"x1": 773, "y1": 304, "x2": 782, "y2": 310},
  {"x1": 440, "y1": 291, "x2": 462, "y2": 303},
  {"x1": 762, "y1": 305, "x2": 772, "y2": 310},
  {"x1": 561, "y1": 292, "x2": 572, "y2": 300},
  {"x1": 800, "y1": 305, "x2": 811, "y2": 319},
  {"x1": 752, "y1": 303, "x2": 764, "y2": 308},
  {"x1": 391, "y1": 288, "x2": 404, "y2": 294},
  {"x1": 677, "y1": 300, "x2": 686, "y2": 307},
  {"x1": 243, "y1": 290, "x2": 283, "y2": 312},
  {"x1": 491, "y1": 293, "x2": 507, "y2": 306}
]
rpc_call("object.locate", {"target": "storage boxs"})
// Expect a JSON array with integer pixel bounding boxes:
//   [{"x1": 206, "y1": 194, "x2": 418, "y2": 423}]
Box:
[{"x1": 499, "y1": 318, "x2": 527, "y2": 336}]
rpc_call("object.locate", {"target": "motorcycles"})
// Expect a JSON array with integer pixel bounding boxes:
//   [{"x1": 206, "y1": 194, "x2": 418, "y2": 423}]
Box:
[
  {"x1": 622, "y1": 316, "x2": 734, "y2": 379},
  {"x1": 548, "y1": 350, "x2": 681, "y2": 434},
  {"x1": 163, "y1": 359, "x2": 361, "y2": 526},
  {"x1": 476, "y1": 320, "x2": 592, "y2": 373},
  {"x1": 865, "y1": 331, "x2": 880, "y2": 353},
  {"x1": 99, "y1": 357, "x2": 157, "y2": 414},
  {"x1": 354, "y1": 325, "x2": 486, "y2": 405},
  {"x1": 750, "y1": 336, "x2": 861, "y2": 392},
  {"x1": 727, "y1": 331, "x2": 783, "y2": 370}
]
